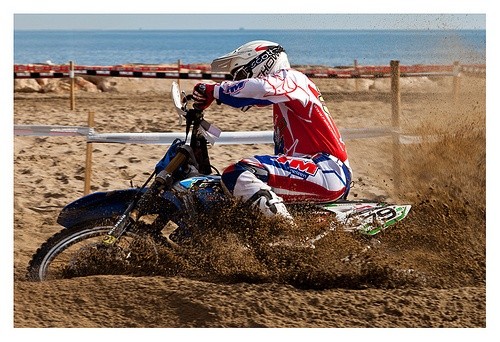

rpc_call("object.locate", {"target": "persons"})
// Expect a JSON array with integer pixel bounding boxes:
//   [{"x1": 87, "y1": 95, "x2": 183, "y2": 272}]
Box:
[{"x1": 182, "y1": 38, "x2": 352, "y2": 253}]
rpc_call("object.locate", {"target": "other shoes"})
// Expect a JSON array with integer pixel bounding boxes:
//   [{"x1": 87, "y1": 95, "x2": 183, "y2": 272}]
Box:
[{"x1": 263, "y1": 234, "x2": 315, "y2": 248}]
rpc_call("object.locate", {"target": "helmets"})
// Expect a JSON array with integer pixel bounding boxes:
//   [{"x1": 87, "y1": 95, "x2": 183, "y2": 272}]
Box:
[{"x1": 211, "y1": 40, "x2": 290, "y2": 79}]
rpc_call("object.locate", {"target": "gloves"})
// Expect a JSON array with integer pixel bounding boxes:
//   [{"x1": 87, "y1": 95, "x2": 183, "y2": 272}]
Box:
[{"x1": 192, "y1": 82, "x2": 214, "y2": 110}]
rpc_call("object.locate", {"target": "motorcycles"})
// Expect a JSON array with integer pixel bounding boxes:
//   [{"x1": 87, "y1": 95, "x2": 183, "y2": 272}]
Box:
[{"x1": 26, "y1": 82, "x2": 413, "y2": 283}]
